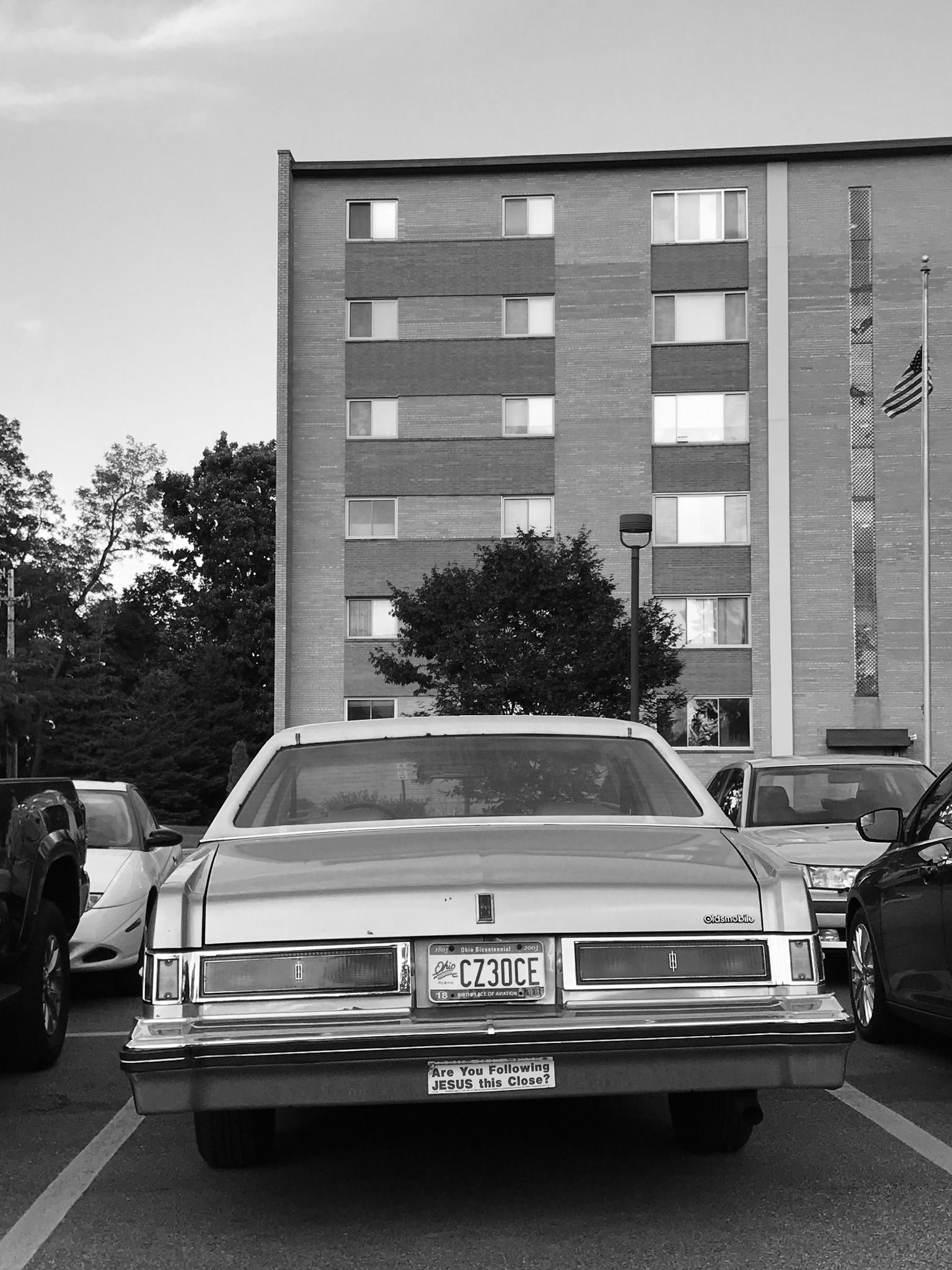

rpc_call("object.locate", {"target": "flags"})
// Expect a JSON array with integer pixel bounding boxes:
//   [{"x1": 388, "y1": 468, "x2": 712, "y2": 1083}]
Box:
[{"x1": 880, "y1": 342, "x2": 934, "y2": 419}]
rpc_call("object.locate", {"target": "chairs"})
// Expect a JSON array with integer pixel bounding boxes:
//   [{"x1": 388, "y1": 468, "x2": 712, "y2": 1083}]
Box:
[{"x1": 758, "y1": 785, "x2": 800, "y2": 827}]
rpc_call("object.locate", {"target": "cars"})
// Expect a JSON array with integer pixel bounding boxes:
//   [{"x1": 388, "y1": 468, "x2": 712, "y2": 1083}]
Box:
[
  {"x1": 0, "y1": 767, "x2": 174, "y2": 1023},
  {"x1": 835, "y1": 762, "x2": 952, "y2": 1053},
  {"x1": 703, "y1": 747, "x2": 943, "y2": 962},
  {"x1": 112, "y1": 713, "x2": 853, "y2": 1173}
]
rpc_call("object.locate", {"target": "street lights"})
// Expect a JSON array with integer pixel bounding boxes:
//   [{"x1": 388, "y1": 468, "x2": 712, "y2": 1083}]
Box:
[{"x1": 619, "y1": 511, "x2": 653, "y2": 730}]
